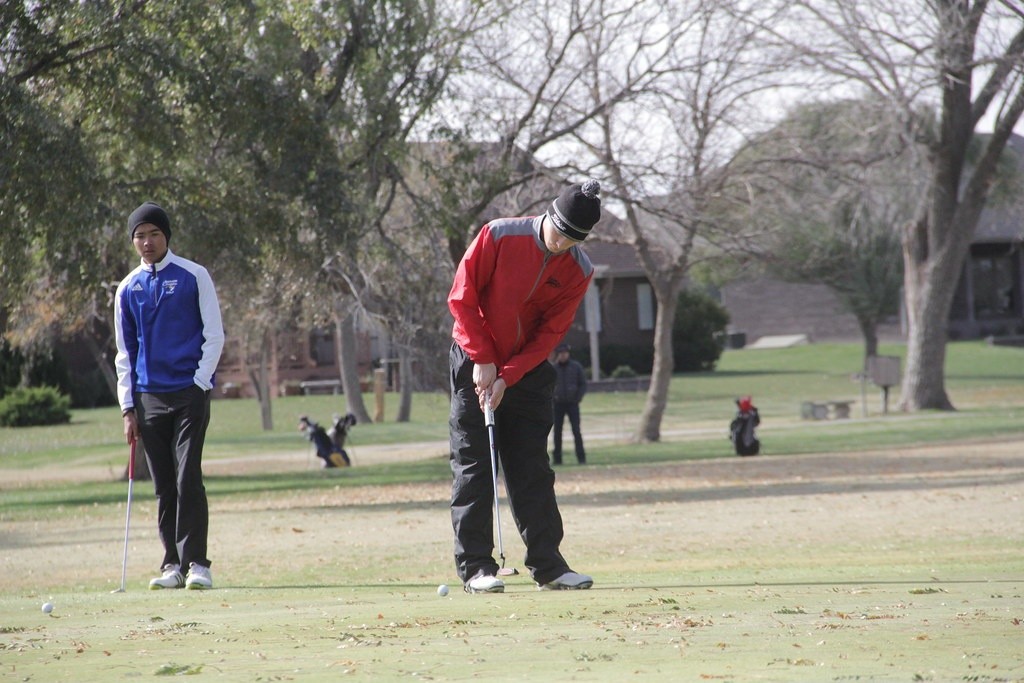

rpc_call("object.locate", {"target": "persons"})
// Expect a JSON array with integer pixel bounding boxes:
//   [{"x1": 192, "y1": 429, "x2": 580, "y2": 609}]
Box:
[
  {"x1": 114, "y1": 202, "x2": 225, "y2": 590},
  {"x1": 444, "y1": 179, "x2": 600, "y2": 592}
]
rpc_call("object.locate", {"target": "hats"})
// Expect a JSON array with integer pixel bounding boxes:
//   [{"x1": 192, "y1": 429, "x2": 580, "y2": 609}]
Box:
[
  {"x1": 129, "y1": 200, "x2": 171, "y2": 244},
  {"x1": 547, "y1": 180, "x2": 601, "y2": 242}
]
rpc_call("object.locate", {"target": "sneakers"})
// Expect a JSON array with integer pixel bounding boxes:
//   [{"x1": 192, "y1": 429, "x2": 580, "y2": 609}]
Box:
[
  {"x1": 537, "y1": 572, "x2": 592, "y2": 590},
  {"x1": 463, "y1": 571, "x2": 503, "y2": 594},
  {"x1": 150, "y1": 565, "x2": 213, "y2": 590}
]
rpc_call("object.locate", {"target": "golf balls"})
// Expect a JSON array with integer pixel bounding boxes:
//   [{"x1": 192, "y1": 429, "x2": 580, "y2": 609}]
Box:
[
  {"x1": 437, "y1": 584, "x2": 449, "y2": 598},
  {"x1": 42, "y1": 603, "x2": 54, "y2": 614}
]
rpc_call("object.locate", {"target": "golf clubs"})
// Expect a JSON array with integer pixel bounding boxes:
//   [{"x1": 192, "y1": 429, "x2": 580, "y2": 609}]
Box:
[
  {"x1": 110, "y1": 432, "x2": 137, "y2": 593},
  {"x1": 484, "y1": 384, "x2": 518, "y2": 576}
]
